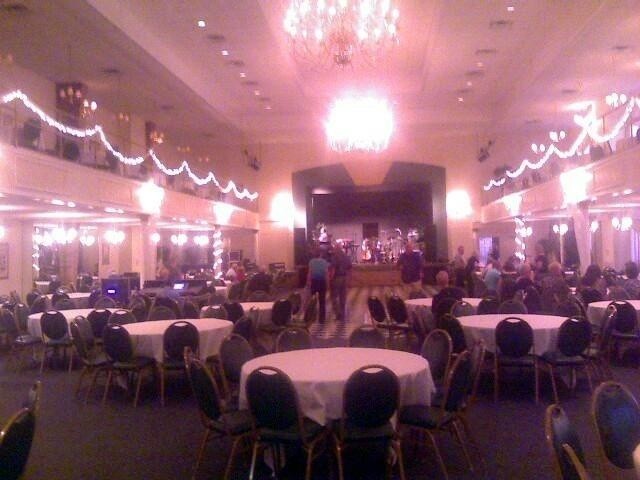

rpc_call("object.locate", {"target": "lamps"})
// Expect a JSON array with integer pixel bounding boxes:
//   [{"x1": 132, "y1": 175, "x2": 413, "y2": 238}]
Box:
[{"x1": 283, "y1": 0, "x2": 400, "y2": 70}]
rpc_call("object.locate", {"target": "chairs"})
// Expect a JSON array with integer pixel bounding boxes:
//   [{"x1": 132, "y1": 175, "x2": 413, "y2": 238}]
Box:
[
  {"x1": 367, "y1": 261, "x2": 640, "y2": 404},
  {"x1": 544, "y1": 404, "x2": 589, "y2": 479},
  {"x1": 185, "y1": 326, "x2": 486, "y2": 480},
  {"x1": 2, "y1": 261, "x2": 320, "y2": 407},
  {"x1": 1, "y1": 409, "x2": 35, "y2": 479},
  {"x1": 593, "y1": 382, "x2": 640, "y2": 469}
]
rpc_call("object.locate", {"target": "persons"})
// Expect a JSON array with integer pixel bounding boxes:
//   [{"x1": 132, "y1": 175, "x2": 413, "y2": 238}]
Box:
[
  {"x1": 225, "y1": 261, "x2": 272, "y2": 301},
  {"x1": 302, "y1": 225, "x2": 352, "y2": 325},
  {"x1": 431, "y1": 238, "x2": 640, "y2": 322},
  {"x1": 397, "y1": 241, "x2": 426, "y2": 298}
]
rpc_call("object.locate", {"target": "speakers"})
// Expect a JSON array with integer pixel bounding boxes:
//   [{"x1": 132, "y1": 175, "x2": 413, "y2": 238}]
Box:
[
  {"x1": 294, "y1": 227, "x2": 305, "y2": 265},
  {"x1": 423, "y1": 224, "x2": 437, "y2": 262}
]
